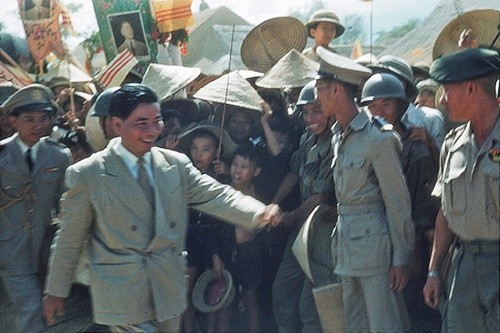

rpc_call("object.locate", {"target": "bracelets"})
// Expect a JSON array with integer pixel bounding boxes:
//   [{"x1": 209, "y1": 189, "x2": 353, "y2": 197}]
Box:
[{"x1": 428, "y1": 272, "x2": 440, "y2": 277}]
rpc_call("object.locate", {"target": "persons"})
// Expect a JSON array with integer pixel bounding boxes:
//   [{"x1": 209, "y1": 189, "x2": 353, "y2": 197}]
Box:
[
  {"x1": 0, "y1": 48, "x2": 339, "y2": 333},
  {"x1": 423, "y1": 29, "x2": 500, "y2": 333},
  {"x1": 303, "y1": 10, "x2": 345, "y2": 63},
  {"x1": 26, "y1": 0, "x2": 49, "y2": 18},
  {"x1": 118, "y1": 21, "x2": 148, "y2": 56},
  {"x1": 41, "y1": 83, "x2": 281, "y2": 333},
  {"x1": 359, "y1": 56, "x2": 449, "y2": 333},
  {"x1": 303, "y1": 46, "x2": 415, "y2": 333}
]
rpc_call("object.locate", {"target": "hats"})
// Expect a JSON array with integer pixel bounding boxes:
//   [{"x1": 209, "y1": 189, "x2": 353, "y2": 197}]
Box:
[
  {"x1": 409, "y1": 64, "x2": 430, "y2": 76},
  {"x1": 428, "y1": 45, "x2": 500, "y2": 84},
  {"x1": 291, "y1": 205, "x2": 341, "y2": 287},
  {"x1": 256, "y1": 46, "x2": 323, "y2": 88},
  {"x1": 240, "y1": 16, "x2": 307, "y2": 74},
  {"x1": 48, "y1": 63, "x2": 94, "y2": 87},
  {"x1": 193, "y1": 70, "x2": 269, "y2": 111},
  {"x1": 432, "y1": 9, "x2": 500, "y2": 56},
  {"x1": 178, "y1": 125, "x2": 238, "y2": 160},
  {"x1": 142, "y1": 60, "x2": 202, "y2": 101},
  {"x1": 0, "y1": 84, "x2": 58, "y2": 116},
  {"x1": 303, "y1": 45, "x2": 373, "y2": 88}
]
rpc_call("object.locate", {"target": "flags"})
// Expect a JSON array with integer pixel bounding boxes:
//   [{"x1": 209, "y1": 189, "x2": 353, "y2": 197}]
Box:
[
  {"x1": 94, "y1": 48, "x2": 140, "y2": 88},
  {"x1": 152, "y1": 0, "x2": 193, "y2": 33}
]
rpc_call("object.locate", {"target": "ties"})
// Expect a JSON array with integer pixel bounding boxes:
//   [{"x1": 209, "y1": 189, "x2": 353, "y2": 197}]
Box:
[
  {"x1": 136, "y1": 158, "x2": 155, "y2": 210},
  {"x1": 130, "y1": 41, "x2": 135, "y2": 56},
  {"x1": 26, "y1": 148, "x2": 33, "y2": 174}
]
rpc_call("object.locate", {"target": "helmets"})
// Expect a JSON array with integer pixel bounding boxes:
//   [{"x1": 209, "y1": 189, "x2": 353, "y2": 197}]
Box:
[
  {"x1": 365, "y1": 54, "x2": 418, "y2": 101},
  {"x1": 90, "y1": 87, "x2": 120, "y2": 116},
  {"x1": 359, "y1": 75, "x2": 409, "y2": 106},
  {"x1": 191, "y1": 268, "x2": 236, "y2": 311},
  {"x1": 303, "y1": 9, "x2": 345, "y2": 39}
]
